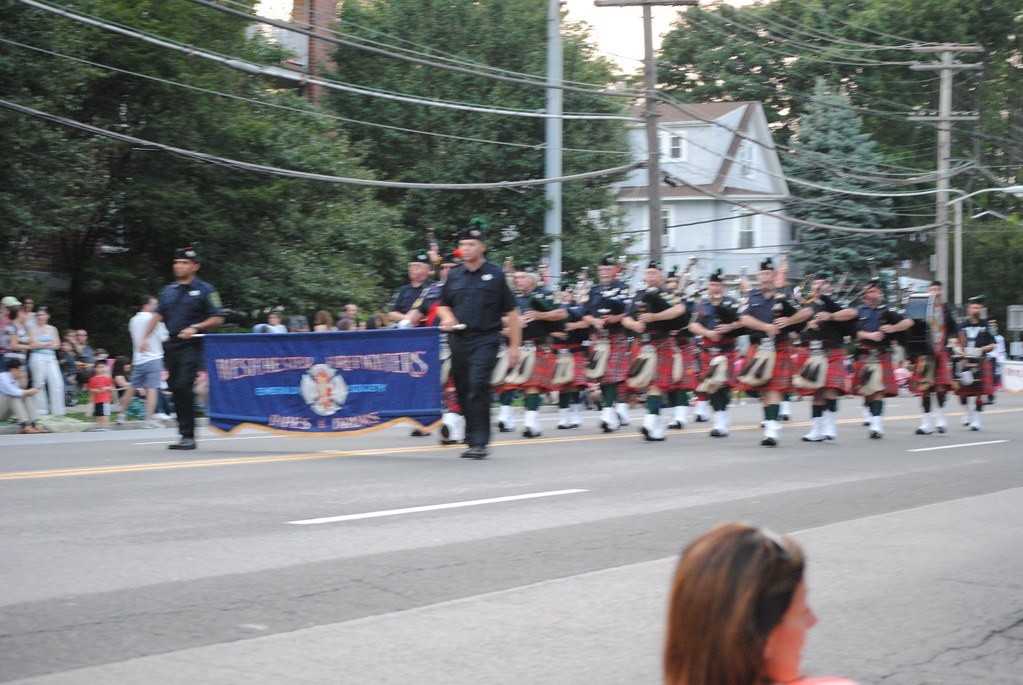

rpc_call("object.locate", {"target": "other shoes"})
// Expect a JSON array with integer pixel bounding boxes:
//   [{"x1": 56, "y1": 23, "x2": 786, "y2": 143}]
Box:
[
  {"x1": 139, "y1": 419, "x2": 166, "y2": 429},
  {"x1": 114, "y1": 414, "x2": 127, "y2": 424},
  {"x1": 19, "y1": 425, "x2": 51, "y2": 434}
]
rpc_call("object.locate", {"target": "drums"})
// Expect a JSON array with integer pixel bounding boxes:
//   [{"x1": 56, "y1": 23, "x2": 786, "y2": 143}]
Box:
[{"x1": 901, "y1": 291, "x2": 947, "y2": 357}]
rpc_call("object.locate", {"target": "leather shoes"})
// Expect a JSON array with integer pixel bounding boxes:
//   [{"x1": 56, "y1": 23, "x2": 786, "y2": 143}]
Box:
[
  {"x1": 460, "y1": 445, "x2": 488, "y2": 459},
  {"x1": 167, "y1": 438, "x2": 197, "y2": 450}
]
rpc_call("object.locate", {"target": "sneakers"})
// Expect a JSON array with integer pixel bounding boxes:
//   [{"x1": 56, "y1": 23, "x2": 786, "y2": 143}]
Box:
[{"x1": 410, "y1": 395, "x2": 997, "y2": 448}]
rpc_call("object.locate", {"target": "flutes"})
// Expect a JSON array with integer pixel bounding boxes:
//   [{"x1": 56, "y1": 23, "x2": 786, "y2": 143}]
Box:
[
  {"x1": 704, "y1": 260, "x2": 750, "y2": 314},
  {"x1": 639, "y1": 256, "x2": 707, "y2": 317},
  {"x1": 745, "y1": 250, "x2": 915, "y2": 318},
  {"x1": 501, "y1": 242, "x2": 641, "y2": 341}
]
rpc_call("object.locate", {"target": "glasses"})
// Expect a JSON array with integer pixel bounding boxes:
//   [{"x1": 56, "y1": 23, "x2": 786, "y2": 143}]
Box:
[
  {"x1": 23, "y1": 302, "x2": 34, "y2": 304},
  {"x1": 35, "y1": 314, "x2": 46, "y2": 316}
]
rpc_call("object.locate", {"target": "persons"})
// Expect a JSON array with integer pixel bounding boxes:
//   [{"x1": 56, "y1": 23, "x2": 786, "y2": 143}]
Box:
[
  {"x1": 139, "y1": 248, "x2": 226, "y2": 451},
  {"x1": 385, "y1": 228, "x2": 1007, "y2": 458},
  {"x1": 662, "y1": 521, "x2": 857, "y2": 685},
  {"x1": 251, "y1": 303, "x2": 390, "y2": 334},
  {"x1": 0, "y1": 295, "x2": 208, "y2": 434}
]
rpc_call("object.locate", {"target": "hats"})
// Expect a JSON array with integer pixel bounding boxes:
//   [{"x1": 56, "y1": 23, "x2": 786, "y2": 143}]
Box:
[
  {"x1": 812, "y1": 270, "x2": 835, "y2": 285},
  {"x1": 865, "y1": 279, "x2": 888, "y2": 290},
  {"x1": 930, "y1": 281, "x2": 942, "y2": 286},
  {"x1": 646, "y1": 260, "x2": 658, "y2": 269},
  {"x1": 968, "y1": 294, "x2": 986, "y2": 305},
  {"x1": 666, "y1": 265, "x2": 678, "y2": 278},
  {"x1": 408, "y1": 251, "x2": 430, "y2": 265},
  {"x1": 599, "y1": 252, "x2": 618, "y2": 265},
  {"x1": 173, "y1": 246, "x2": 198, "y2": 260},
  {"x1": 0, "y1": 296, "x2": 22, "y2": 307},
  {"x1": 513, "y1": 261, "x2": 538, "y2": 273},
  {"x1": 456, "y1": 227, "x2": 484, "y2": 241},
  {"x1": 759, "y1": 257, "x2": 775, "y2": 270},
  {"x1": 710, "y1": 268, "x2": 723, "y2": 283}
]
